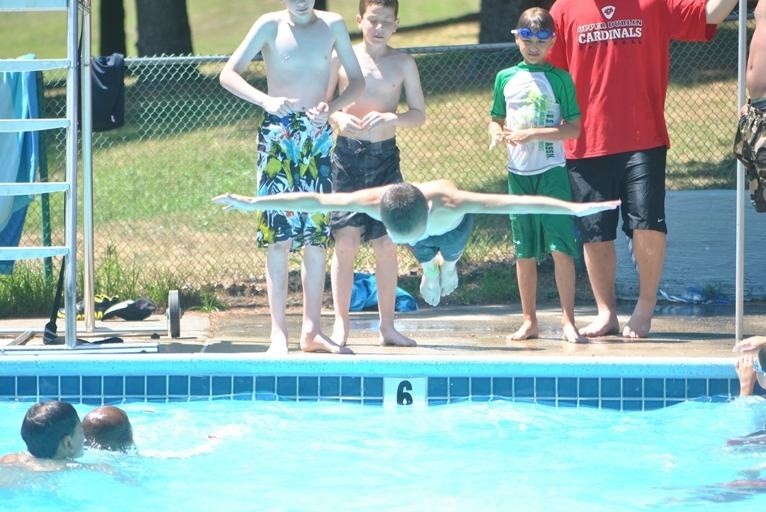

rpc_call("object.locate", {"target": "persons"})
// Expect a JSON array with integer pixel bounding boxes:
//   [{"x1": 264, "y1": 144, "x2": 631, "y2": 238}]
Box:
[
  {"x1": 734, "y1": 0, "x2": 765, "y2": 216},
  {"x1": 327, "y1": 1, "x2": 428, "y2": 347},
  {"x1": 487, "y1": 8, "x2": 586, "y2": 342},
  {"x1": 212, "y1": 179, "x2": 622, "y2": 308},
  {"x1": 0, "y1": 399, "x2": 113, "y2": 473},
  {"x1": 542, "y1": 0, "x2": 740, "y2": 338},
  {"x1": 218, "y1": 1, "x2": 366, "y2": 355},
  {"x1": 83, "y1": 406, "x2": 135, "y2": 454}
]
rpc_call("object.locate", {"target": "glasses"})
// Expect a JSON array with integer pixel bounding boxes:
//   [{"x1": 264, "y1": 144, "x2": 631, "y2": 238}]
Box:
[{"x1": 511, "y1": 27, "x2": 556, "y2": 39}]
